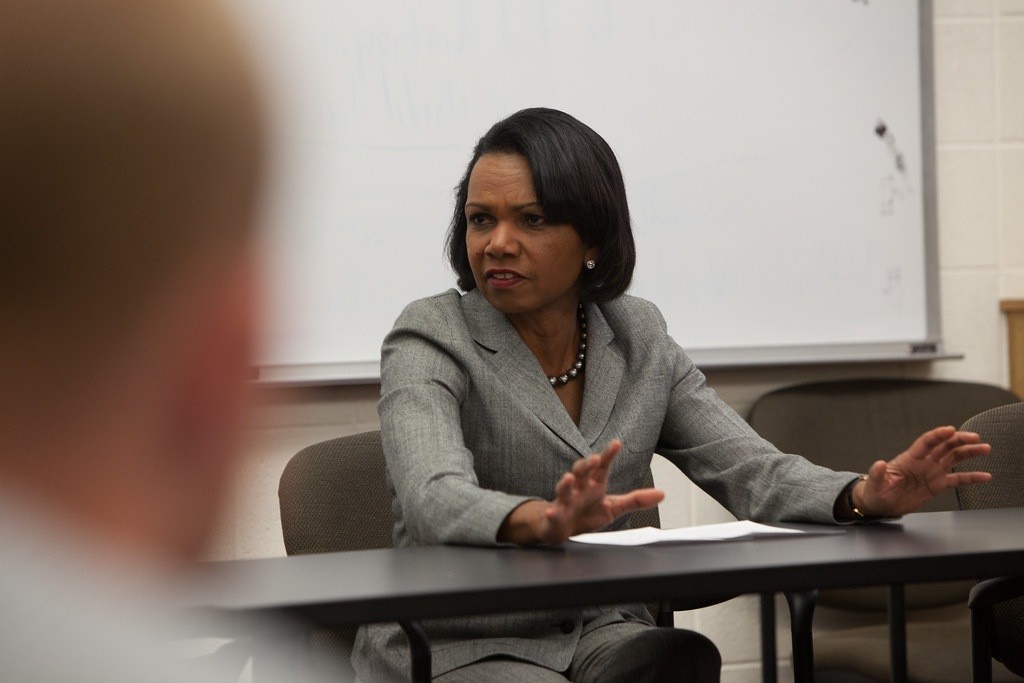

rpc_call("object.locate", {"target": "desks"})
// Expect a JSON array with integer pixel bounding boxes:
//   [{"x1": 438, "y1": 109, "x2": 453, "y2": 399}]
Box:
[{"x1": 184, "y1": 507, "x2": 1024, "y2": 683}]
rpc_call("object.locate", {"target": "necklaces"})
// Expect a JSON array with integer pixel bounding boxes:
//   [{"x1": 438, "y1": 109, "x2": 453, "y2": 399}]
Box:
[{"x1": 547, "y1": 303, "x2": 588, "y2": 387}]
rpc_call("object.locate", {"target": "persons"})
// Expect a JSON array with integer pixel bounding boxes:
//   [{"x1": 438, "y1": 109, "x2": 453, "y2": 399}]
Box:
[
  {"x1": 0, "y1": 0, "x2": 363, "y2": 683},
  {"x1": 347, "y1": 108, "x2": 993, "y2": 683}
]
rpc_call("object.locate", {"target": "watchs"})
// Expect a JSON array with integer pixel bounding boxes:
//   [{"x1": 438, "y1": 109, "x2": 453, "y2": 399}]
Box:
[{"x1": 844, "y1": 473, "x2": 880, "y2": 520}]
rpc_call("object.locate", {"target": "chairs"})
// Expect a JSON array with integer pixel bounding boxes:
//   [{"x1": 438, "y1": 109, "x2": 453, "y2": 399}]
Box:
[
  {"x1": 745, "y1": 378, "x2": 1024, "y2": 683},
  {"x1": 214, "y1": 430, "x2": 675, "y2": 683},
  {"x1": 952, "y1": 402, "x2": 1024, "y2": 683}
]
